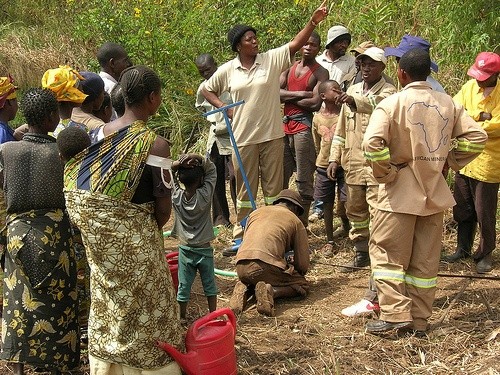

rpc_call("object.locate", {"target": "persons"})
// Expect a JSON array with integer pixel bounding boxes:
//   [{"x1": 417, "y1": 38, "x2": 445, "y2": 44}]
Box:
[
  {"x1": 340, "y1": 35, "x2": 447, "y2": 317},
  {"x1": 349, "y1": 41, "x2": 396, "y2": 87},
  {"x1": 362, "y1": 49, "x2": 489, "y2": 335},
  {"x1": 228, "y1": 189, "x2": 310, "y2": 317},
  {"x1": 326, "y1": 49, "x2": 396, "y2": 270},
  {"x1": 315, "y1": 25, "x2": 358, "y2": 110},
  {"x1": 170, "y1": 155, "x2": 218, "y2": 326},
  {"x1": 312, "y1": 80, "x2": 353, "y2": 257},
  {"x1": 97, "y1": 43, "x2": 130, "y2": 122},
  {"x1": 57, "y1": 66, "x2": 187, "y2": 375},
  {"x1": 444, "y1": 52, "x2": 499, "y2": 274},
  {"x1": 58, "y1": 126, "x2": 116, "y2": 233},
  {"x1": 194, "y1": 53, "x2": 239, "y2": 227},
  {"x1": 0, "y1": 65, "x2": 125, "y2": 375},
  {"x1": 202, "y1": 0, "x2": 328, "y2": 256}
]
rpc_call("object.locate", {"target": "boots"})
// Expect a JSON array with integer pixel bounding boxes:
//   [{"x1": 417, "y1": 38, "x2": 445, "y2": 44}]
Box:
[
  {"x1": 366, "y1": 319, "x2": 411, "y2": 332},
  {"x1": 353, "y1": 240, "x2": 370, "y2": 267}
]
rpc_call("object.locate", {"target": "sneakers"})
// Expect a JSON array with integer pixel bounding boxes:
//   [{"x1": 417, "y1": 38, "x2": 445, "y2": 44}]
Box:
[
  {"x1": 342, "y1": 300, "x2": 380, "y2": 317},
  {"x1": 308, "y1": 212, "x2": 323, "y2": 222}
]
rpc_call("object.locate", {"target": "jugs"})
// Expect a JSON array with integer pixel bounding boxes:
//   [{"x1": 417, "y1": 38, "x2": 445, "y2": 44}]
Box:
[
  {"x1": 166, "y1": 251, "x2": 178, "y2": 291},
  {"x1": 156, "y1": 308, "x2": 238, "y2": 375}
]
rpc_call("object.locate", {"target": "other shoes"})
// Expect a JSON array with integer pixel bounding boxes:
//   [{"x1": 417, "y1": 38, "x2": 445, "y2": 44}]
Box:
[
  {"x1": 222, "y1": 246, "x2": 237, "y2": 256},
  {"x1": 477, "y1": 254, "x2": 492, "y2": 272},
  {"x1": 323, "y1": 243, "x2": 337, "y2": 256},
  {"x1": 255, "y1": 281, "x2": 275, "y2": 316},
  {"x1": 230, "y1": 281, "x2": 250, "y2": 313},
  {"x1": 444, "y1": 253, "x2": 470, "y2": 262}
]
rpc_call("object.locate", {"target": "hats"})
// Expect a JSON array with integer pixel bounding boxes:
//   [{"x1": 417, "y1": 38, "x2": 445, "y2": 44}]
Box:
[
  {"x1": 357, "y1": 47, "x2": 387, "y2": 65},
  {"x1": 273, "y1": 189, "x2": 306, "y2": 216},
  {"x1": 325, "y1": 26, "x2": 351, "y2": 48},
  {"x1": 384, "y1": 34, "x2": 438, "y2": 73},
  {"x1": 228, "y1": 24, "x2": 256, "y2": 52},
  {"x1": 350, "y1": 41, "x2": 375, "y2": 54},
  {"x1": 467, "y1": 51, "x2": 500, "y2": 81}
]
